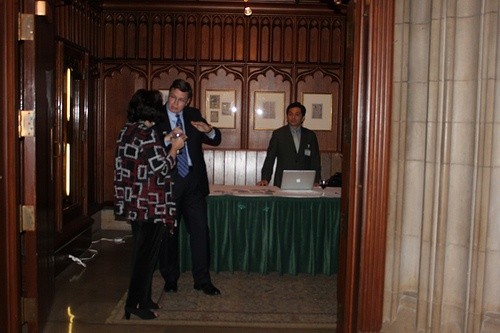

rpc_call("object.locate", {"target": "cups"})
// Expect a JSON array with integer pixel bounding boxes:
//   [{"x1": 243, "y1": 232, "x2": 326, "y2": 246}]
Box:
[{"x1": 176, "y1": 133, "x2": 182, "y2": 154}]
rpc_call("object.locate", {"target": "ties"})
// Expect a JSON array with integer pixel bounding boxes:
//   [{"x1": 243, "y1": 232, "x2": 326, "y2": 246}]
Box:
[{"x1": 175, "y1": 114, "x2": 190, "y2": 179}]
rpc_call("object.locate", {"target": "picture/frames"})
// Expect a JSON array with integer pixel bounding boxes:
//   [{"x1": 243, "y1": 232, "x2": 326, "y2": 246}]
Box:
[
  {"x1": 158, "y1": 88, "x2": 170, "y2": 105},
  {"x1": 205, "y1": 89, "x2": 236, "y2": 130},
  {"x1": 253, "y1": 91, "x2": 286, "y2": 131},
  {"x1": 300, "y1": 92, "x2": 334, "y2": 132}
]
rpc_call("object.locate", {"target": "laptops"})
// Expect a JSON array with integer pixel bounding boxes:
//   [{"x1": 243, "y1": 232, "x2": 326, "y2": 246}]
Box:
[{"x1": 280, "y1": 170, "x2": 317, "y2": 191}]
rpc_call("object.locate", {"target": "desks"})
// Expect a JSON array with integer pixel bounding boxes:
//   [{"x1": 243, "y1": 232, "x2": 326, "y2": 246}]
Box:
[{"x1": 179, "y1": 185, "x2": 341, "y2": 277}]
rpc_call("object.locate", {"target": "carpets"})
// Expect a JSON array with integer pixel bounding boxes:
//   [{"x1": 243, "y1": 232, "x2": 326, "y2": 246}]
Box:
[{"x1": 104, "y1": 268, "x2": 338, "y2": 329}]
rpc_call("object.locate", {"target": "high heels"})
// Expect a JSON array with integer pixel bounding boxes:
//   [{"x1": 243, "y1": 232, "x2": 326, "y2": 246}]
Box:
[
  {"x1": 139, "y1": 301, "x2": 158, "y2": 308},
  {"x1": 124, "y1": 305, "x2": 155, "y2": 320}
]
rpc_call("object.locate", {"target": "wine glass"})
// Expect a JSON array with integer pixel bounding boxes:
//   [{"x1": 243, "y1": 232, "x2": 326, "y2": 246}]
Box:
[{"x1": 319, "y1": 179, "x2": 328, "y2": 197}]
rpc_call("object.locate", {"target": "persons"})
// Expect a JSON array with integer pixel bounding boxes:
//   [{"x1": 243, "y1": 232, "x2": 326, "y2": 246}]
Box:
[
  {"x1": 157, "y1": 80, "x2": 221, "y2": 295},
  {"x1": 257, "y1": 103, "x2": 321, "y2": 186},
  {"x1": 114, "y1": 89, "x2": 188, "y2": 319}
]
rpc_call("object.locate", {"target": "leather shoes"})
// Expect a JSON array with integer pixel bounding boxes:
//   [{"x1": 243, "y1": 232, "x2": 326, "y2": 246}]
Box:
[
  {"x1": 164, "y1": 283, "x2": 178, "y2": 292},
  {"x1": 194, "y1": 282, "x2": 220, "y2": 295}
]
